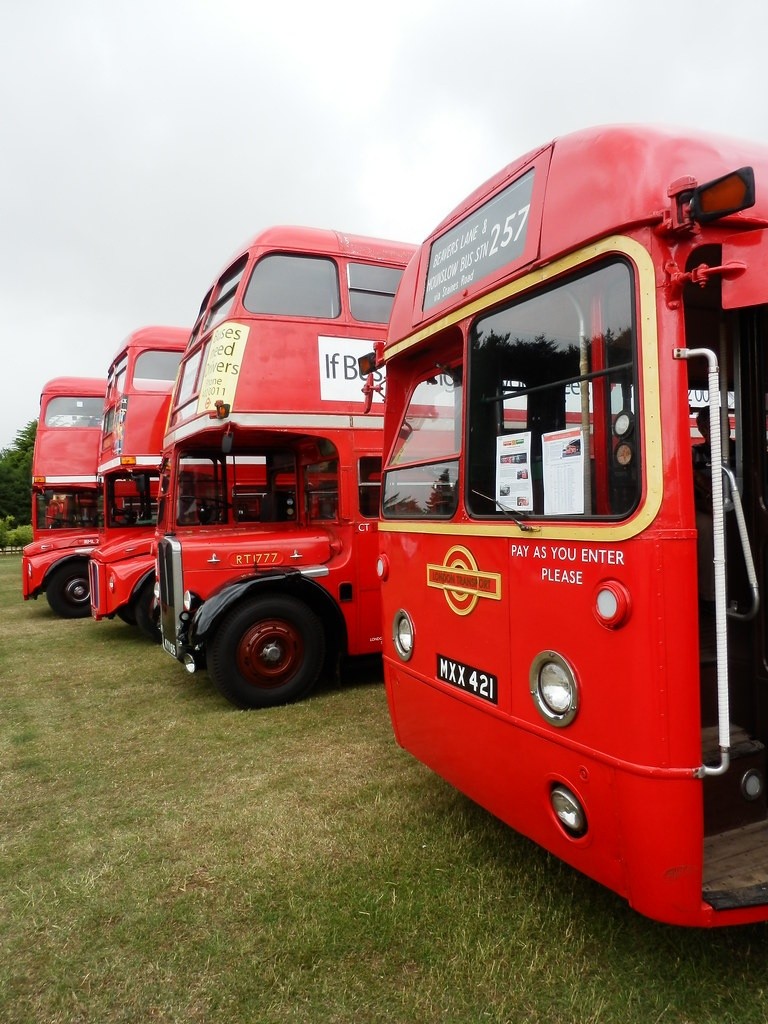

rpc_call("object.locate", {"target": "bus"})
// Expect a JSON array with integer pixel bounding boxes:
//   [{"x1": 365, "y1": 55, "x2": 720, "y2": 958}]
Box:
[
  {"x1": 19, "y1": 324, "x2": 382, "y2": 642},
  {"x1": 355, "y1": 122, "x2": 768, "y2": 931},
  {"x1": 141, "y1": 224, "x2": 736, "y2": 709}
]
